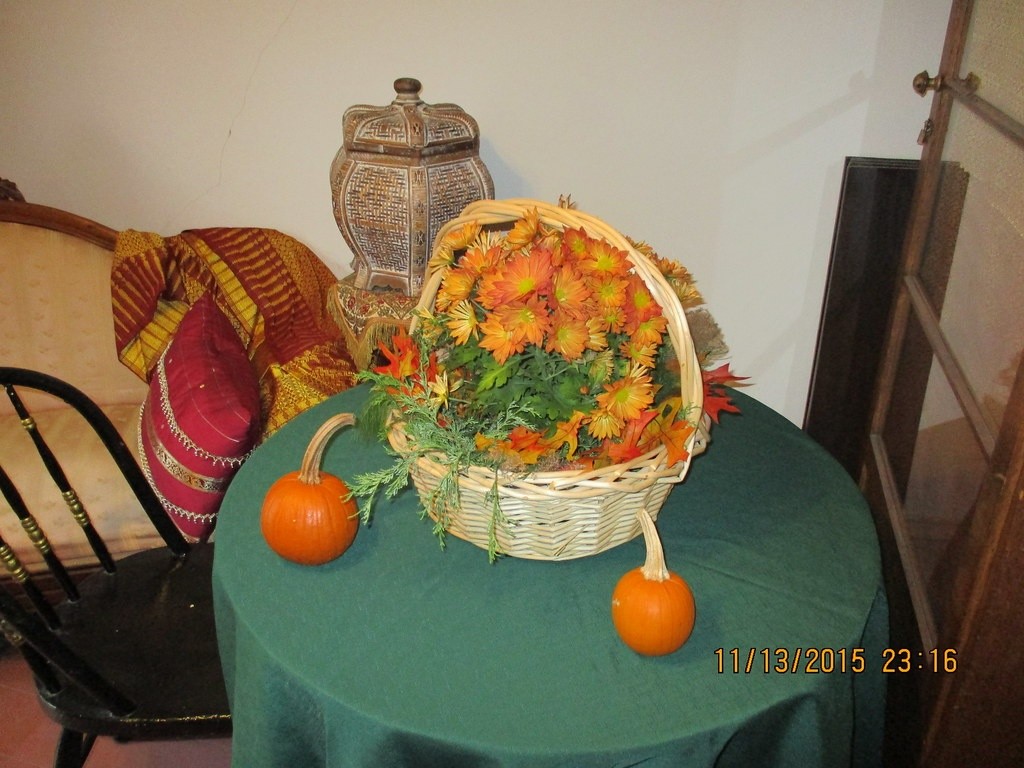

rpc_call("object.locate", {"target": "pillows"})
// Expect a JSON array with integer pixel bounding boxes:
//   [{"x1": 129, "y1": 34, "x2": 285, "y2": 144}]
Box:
[{"x1": 138, "y1": 289, "x2": 265, "y2": 543}]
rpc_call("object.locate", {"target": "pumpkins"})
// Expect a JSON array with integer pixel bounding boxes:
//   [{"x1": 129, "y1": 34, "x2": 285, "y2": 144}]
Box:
[
  {"x1": 262, "y1": 413, "x2": 359, "y2": 565},
  {"x1": 611, "y1": 509, "x2": 697, "y2": 656}
]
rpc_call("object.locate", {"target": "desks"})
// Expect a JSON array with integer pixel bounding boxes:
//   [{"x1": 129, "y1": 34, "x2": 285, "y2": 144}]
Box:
[{"x1": 213, "y1": 380, "x2": 892, "y2": 768}]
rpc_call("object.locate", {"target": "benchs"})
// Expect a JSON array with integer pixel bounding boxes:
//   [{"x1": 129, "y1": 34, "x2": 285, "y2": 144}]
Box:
[{"x1": 0, "y1": 200, "x2": 358, "y2": 580}]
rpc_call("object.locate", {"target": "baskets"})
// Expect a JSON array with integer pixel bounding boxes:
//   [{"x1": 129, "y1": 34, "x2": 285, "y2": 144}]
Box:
[{"x1": 385, "y1": 198, "x2": 711, "y2": 562}]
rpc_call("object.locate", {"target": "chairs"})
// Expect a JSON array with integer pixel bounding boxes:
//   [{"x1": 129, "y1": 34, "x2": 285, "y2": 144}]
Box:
[{"x1": 0, "y1": 367, "x2": 232, "y2": 768}]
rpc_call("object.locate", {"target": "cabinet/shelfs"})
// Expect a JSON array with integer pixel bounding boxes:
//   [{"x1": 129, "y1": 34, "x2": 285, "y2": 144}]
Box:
[{"x1": 854, "y1": 0, "x2": 1024, "y2": 768}]
